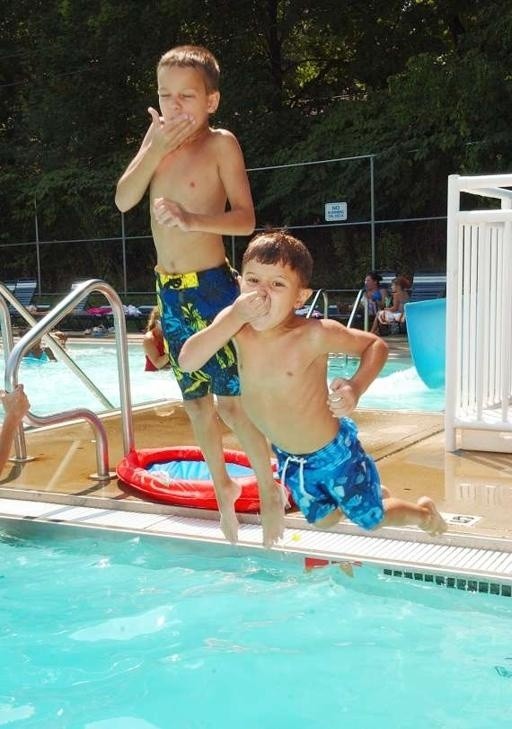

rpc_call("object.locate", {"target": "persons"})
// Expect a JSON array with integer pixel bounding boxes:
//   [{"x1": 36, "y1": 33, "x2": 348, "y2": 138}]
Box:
[
  {"x1": 367, "y1": 275, "x2": 411, "y2": 334},
  {"x1": 332, "y1": 272, "x2": 390, "y2": 316},
  {"x1": 17, "y1": 327, "x2": 68, "y2": 366},
  {"x1": 176, "y1": 222, "x2": 451, "y2": 538},
  {"x1": 0, "y1": 383, "x2": 31, "y2": 479},
  {"x1": 141, "y1": 304, "x2": 174, "y2": 374},
  {"x1": 113, "y1": 43, "x2": 289, "y2": 551}
]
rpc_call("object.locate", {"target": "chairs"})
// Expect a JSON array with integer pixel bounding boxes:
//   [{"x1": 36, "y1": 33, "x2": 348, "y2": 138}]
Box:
[
  {"x1": 3, "y1": 277, "x2": 158, "y2": 332},
  {"x1": 310, "y1": 273, "x2": 446, "y2": 339}
]
